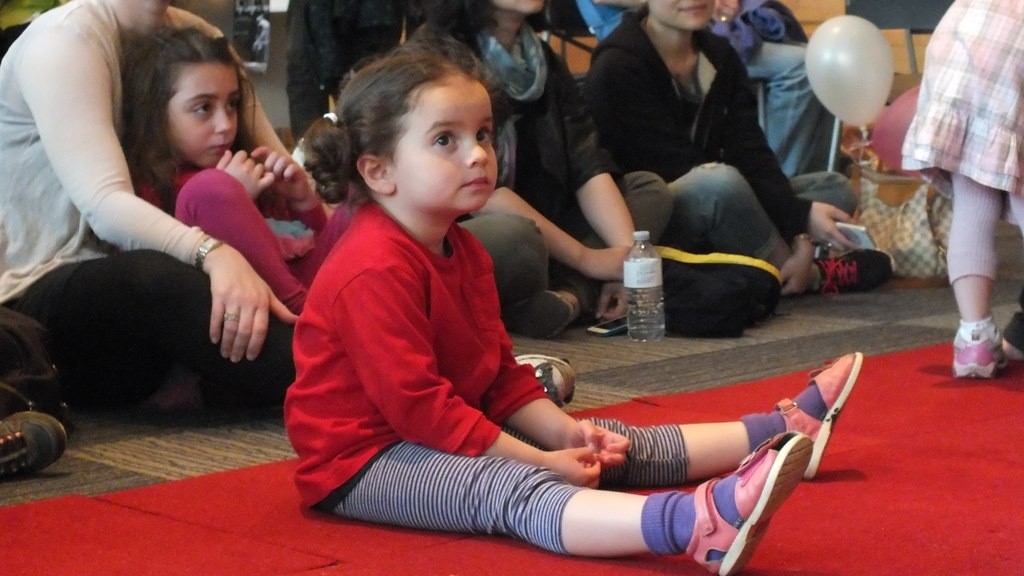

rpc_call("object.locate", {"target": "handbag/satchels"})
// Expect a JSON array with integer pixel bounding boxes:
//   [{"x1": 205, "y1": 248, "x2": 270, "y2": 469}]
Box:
[
  {"x1": 848, "y1": 167, "x2": 952, "y2": 288},
  {"x1": 0, "y1": 305, "x2": 74, "y2": 435},
  {"x1": 656, "y1": 246, "x2": 783, "y2": 340}
]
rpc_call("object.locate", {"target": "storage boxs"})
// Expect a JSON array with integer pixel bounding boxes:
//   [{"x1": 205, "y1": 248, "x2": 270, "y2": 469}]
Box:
[{"x1": 846, "y1": 164, "x2": 930, "y2": 209}]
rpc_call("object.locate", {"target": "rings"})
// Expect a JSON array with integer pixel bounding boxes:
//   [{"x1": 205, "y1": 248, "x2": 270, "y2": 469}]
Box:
[
  {"x1": 827, "y1": 243, "x2": 832, "y2": 248},
  {"x1": 222, "y1": 313, "x2": 241, "y2": 321}
]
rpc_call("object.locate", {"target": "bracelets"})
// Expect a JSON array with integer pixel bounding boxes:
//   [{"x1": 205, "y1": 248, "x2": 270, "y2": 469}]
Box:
[{"x1": 792, "y1": 234, "x2": 810, "y2": 246}]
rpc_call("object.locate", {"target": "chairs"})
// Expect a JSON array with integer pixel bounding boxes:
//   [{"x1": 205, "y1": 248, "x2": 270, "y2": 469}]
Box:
[{"x1": 844, "y1": 1, "x2": 955, "y2": 73}]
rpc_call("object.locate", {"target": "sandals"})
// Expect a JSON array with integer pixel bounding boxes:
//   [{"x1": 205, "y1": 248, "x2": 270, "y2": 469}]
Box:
[
  {"x1": 773, "y1": 354, "x2": 865, "y2": 482},
  {"x1": 686, "y1": 431, "x2": 812, "y2": 576}
]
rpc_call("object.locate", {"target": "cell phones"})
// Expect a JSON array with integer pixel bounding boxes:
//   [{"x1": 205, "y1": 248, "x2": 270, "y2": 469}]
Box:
[
  {"x1": 835, "y1": 221, "x2": 877, "y2": 250},
  {"x1": 586, "y1": 314, "x2": 630, "y2": 336}
]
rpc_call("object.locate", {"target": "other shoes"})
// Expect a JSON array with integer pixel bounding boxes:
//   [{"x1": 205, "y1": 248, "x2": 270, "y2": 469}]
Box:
[
  {"x1": 1002, "y1": 313, "x2": 1024, "y2": 362},
  {"x1": 501, "y1": 290, "x2": 576, "y2": 339},
  {"x1": 513, "y1": 355, "x2": 574, "y2": 407}
]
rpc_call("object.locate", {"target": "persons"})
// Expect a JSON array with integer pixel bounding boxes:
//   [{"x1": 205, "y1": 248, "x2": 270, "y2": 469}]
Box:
[
  {"x1": 0, "y1": 305, "x2": 78, "y2": 482},
  {"x1": 587, "y1": 0, "x2": 895, "y2": 298},
  {"x1": 283, "y1": 20, "x2": 864, "y2": 576},
  {"x1": 405, "y1": 1, "x2": 673, "y2": 340},
  {"x1": 901, "y1": 0, "x2": 1024, "y2": 381},
  {"x1": 283, "y1": 0, "x2": 472, "y2": 145},
  {"x1": 1, "y1": 0, "x2": 336, "y2": 422},
  {"x1": 577, "y1": 1, "x2": 844, "y2": 180},
  {"x1": 117, "y1": 25, "x2": 579, "y2": 410}
]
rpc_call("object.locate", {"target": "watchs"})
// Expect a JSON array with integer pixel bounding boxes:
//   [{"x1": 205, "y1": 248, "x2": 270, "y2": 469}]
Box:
[{"x1": 195, "y1": 236, "x2": 224, "y2": 271}]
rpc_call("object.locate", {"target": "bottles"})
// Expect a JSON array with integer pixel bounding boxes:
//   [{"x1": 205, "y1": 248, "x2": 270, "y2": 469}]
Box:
[{"x1": 623, "y1": 231, "x2": 665, "y2": 342}]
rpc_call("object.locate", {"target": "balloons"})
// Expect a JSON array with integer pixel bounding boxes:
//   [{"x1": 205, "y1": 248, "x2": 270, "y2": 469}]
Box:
[
  {"x1": 804, "y1": 14, "x2": 892, "y2": 129},
  {"x1": 869, "y1": 84, "x2": 925, "y2": 179}
]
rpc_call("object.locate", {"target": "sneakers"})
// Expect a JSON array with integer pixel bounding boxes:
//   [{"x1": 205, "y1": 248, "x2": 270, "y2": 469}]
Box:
[
  {"x1": 954, "y1": 329, "x2": 1009, "y2": 381},
  {"x1": 813, "y1": 247, "x2": 896, "y2": 297},
  {"x1": 0, "y1": 408, "x2": 68, "y2": 483}
]
